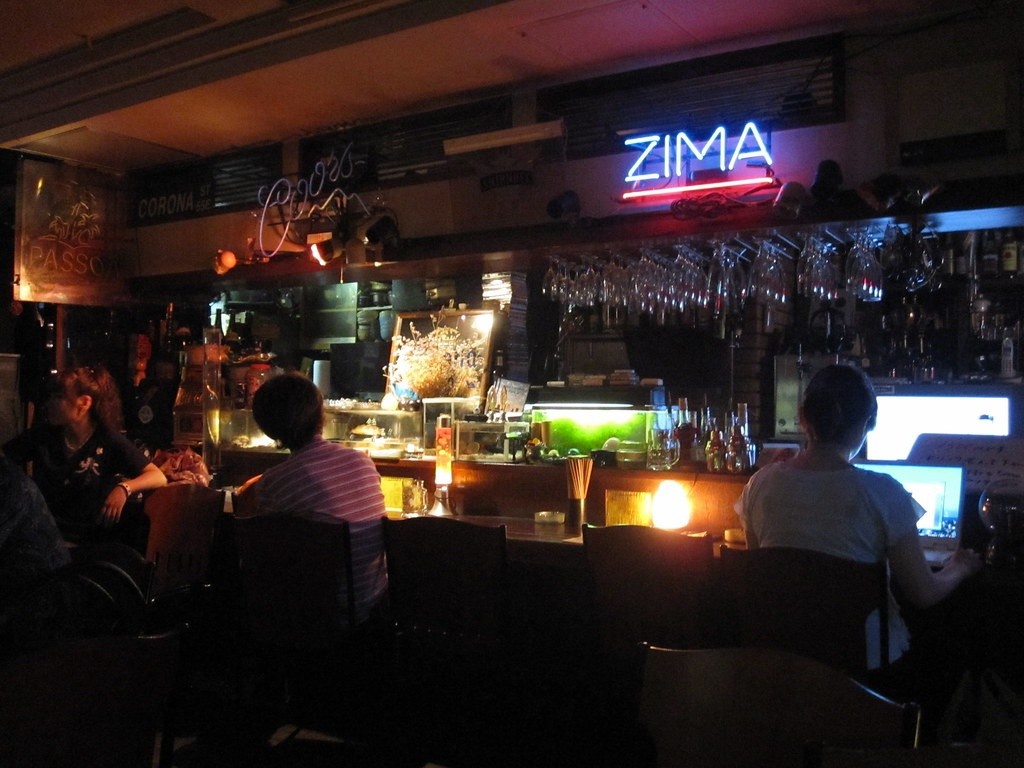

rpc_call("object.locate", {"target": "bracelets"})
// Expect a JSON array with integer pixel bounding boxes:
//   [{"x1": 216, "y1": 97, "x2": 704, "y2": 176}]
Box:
[{"x1": 117, "y1": 482, "x2": 132, "y2": 498}]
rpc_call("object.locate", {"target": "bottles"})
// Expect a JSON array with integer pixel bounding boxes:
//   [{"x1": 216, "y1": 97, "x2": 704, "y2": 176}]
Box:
[
  {"x1": 234, "y1": 382, "x2": 244, "y2": 409},
  {"x1": 492, "y1": 349, "x2": 507, "y2": 382},
  {"x1": 672, "y1": 397, "x2": 756, "y2": 474},
  {"x1": 939, "y1": 226, "x2": 1020, "y2": 283}
]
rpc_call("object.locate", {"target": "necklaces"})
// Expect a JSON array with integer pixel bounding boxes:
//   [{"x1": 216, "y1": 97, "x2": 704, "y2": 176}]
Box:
[{"x1": 63, "y1": 426, "x2": 96, "y2": 451}]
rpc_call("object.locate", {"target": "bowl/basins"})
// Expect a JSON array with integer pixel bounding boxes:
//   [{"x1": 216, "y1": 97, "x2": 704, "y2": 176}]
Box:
[
  {"x1": 370, "y1": 291, "x2": 388, "y2": 305},
  {"x1": 357, "y1": 324, "x2": 377, "y2": 344},
  {"x1": 354, "y1": 310, "x2": 378, "y2": 324},
  {"x1": 379, "y1": 309, "x2": 396, "y2": 343}
]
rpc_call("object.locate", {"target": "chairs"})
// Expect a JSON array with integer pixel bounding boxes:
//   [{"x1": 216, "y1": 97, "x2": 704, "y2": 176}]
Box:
[{"x1": 0, "y1": 483, "x2": 1024, "y2": 768}]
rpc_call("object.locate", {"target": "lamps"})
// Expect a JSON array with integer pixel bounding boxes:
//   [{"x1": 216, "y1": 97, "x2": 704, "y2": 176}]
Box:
[
  {"x1": 366, "y1": 217, "x2": 395, "y2": 245},
  {"x1": 858, "y1": 171, "x2": 907, "y2": 211},
  {"x1": 810, "y1": 160, "x2": 845, "y2": 203},
  {"x1": 547, "y1": 189, "x2": 580, "y2": 219},
  {"x1": 215, "y1": 250, "x2": 237, "y2": 275},
  {"x1": 310, "y1": 236, "x2": 344, "y2": 267},
  {"x1": 772, "y1": 182, "x2": 805, "y2": 218}
]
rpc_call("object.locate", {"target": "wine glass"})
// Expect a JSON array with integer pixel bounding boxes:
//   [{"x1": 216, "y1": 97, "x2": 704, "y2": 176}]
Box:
[{"x1": 541, "y1": 226, "x2": 883, "y2": 315}]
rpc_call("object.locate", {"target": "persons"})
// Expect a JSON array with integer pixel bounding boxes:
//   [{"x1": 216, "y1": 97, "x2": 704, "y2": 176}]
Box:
[
  {"x1": 0, "y1": 363, "x2": 170, "y2": 639},
  {"x1": 0, "y1": 452, "x2": 83, "y2": 665},
  {"x1": 737, "y1": 364, "x2": 985, "y2": 749},
  {"x1": 231, "y1": 374, "x2": 389, "y2": 634}
]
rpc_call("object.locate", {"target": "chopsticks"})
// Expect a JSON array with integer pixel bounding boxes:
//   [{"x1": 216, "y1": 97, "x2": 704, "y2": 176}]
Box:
[{"x1": 565, "y1": 457, "x2": 593, "y2": 499}]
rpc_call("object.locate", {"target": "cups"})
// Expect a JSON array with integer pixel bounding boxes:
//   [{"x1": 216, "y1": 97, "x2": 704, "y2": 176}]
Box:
[
  {"x1": 403, "y1": 436, "x2": 423, "y2": 458},
  {"x1": 645, "y1": 429, "x2": 680, "y2": 471},
  {"x1": 402, "y1": 479, "x2": 427, "y2": 518},
  {"x1": 246, "y1": 363, "x2": 273, "y2": 409},
  {"x1": 312, "y1": 360, "x2": 331, "y2": 399},
  {"x1": 564, "y1": 497, "x2": 585, "y2": 539},
  {"x1": 358, "y1": 295, "x2": 369, "y2": 307}
]
